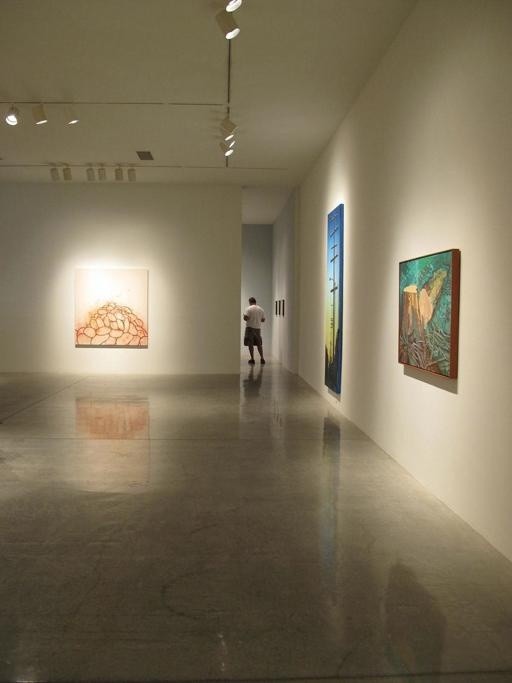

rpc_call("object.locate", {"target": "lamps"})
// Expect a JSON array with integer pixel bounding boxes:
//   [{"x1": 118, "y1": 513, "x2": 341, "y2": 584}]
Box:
[
  {"x1": 218, "y1": 0, "x2": 241, "y2": 157},
  {"x1": 49, "y1": 162, "x2": 137, "y2": 182},
  {"x1": 3, "y1": 101, "x2": 48, "y2": 127}
]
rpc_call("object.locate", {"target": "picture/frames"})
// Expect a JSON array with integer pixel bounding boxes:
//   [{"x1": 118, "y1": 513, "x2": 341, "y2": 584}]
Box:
[{"x1": 398, "y1": 247, "x2": 460, "y2": 379}]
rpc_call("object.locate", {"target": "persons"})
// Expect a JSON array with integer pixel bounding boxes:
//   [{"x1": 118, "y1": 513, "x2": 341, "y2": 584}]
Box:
[{"x1": 241, "y1": 296, "x2": 267, "y2": 366}]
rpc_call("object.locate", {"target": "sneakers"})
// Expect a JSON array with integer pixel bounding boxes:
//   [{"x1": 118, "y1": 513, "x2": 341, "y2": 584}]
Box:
[
  {"x1": 247, "y1": 359, "x2": 256, "y2": 364},
  {"x1": 259, "y1": 358, "x2": 266, "y2": 363}
]
